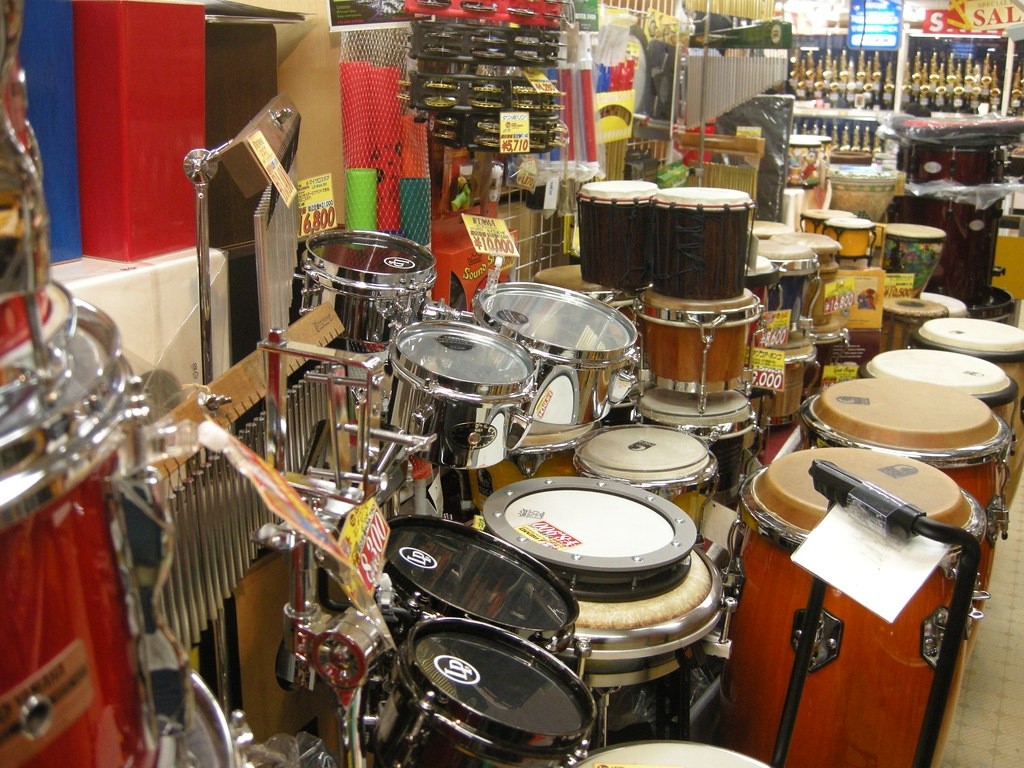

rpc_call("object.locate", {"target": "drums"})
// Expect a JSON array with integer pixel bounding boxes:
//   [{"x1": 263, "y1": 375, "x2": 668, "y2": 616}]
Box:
[
  {"x1": 804, "y1": 375, "x2": 1008, "y2": 679},
  {"x1": 470, "y1": 281, "x2": 641, "y2": 425},
  {"x1": 729, "y1": 450, "x2": 992, "y2": 767},
  {"x1": 575, "y1": 176, "x2": 758, "y2": 495},
  {"x1": 0, "y1": 1, "x2": 272, "y2": 767},
  {"x1": 484, "y1": 426, "x2": 775, "y2": 768},
  {"x1": 536, "y1": 113, "x2": 1024, "y2": 435},
  {"x1": 372, "y1": 510, "x2": 582, "y2": 658},
  {"x1": 342, "y1": 609, "x2": 596, "y2": 767},
  {"x1": 384, "y1": 319, "x2": 539, "y2": 468},
  {"x1": 865, "y1": 342, "x2": 1016, "y2": 500},
  {"x1": 297, "y1": 223, "x2": 431, "y2": 354},
  {"x1": 915, "y1": 317, "x2": 1024, "y2": 516}
]
